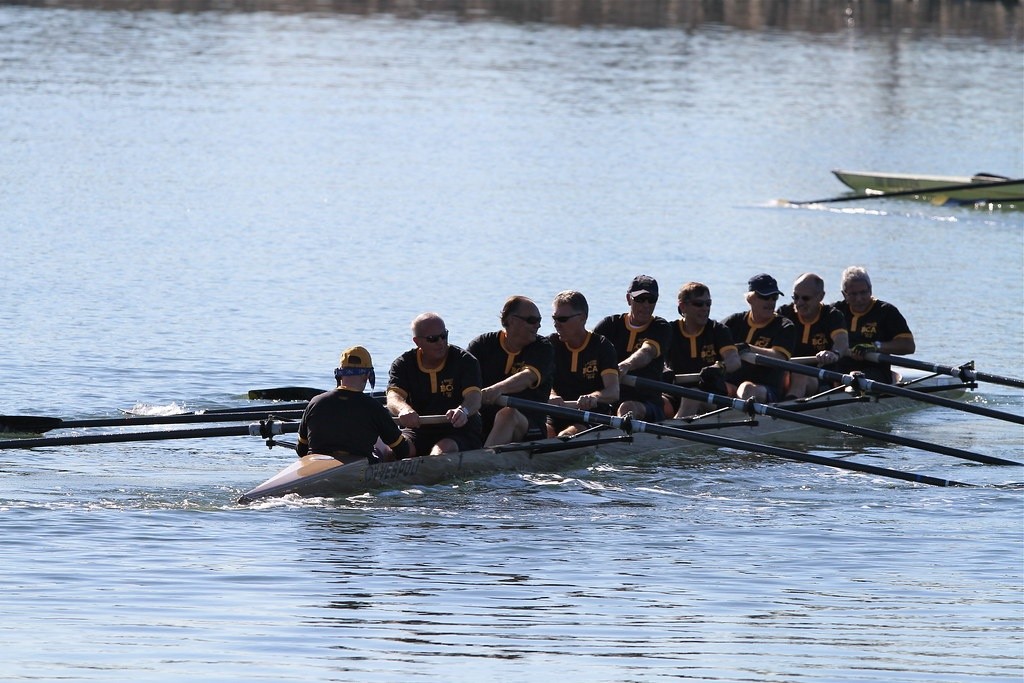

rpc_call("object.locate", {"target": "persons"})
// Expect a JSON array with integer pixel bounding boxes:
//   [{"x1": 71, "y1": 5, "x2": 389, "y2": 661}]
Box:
[
  {"x1": 662, "y1": 281, "x2": 741, "y2": 419},
  {"x1": 592, "y1": 275, "x2": 672, "y2": 419},
  {"x1": 718, "y1": 272, "x2": 795, "y2": 403},
  {"x1": 829, "y1": 266, "x2": 916, "y2": 384},
  {"x1": 465, "y1": 295, "x2": 554, "y2": 448},
  {"x1": 296, "y1": 346, "x2": 410, "y2": 465},
  {"x1": 382, "y1": 312, "x2": 482, "y2": 459},
  {"x1": 776, "y1": 273, "x2": 849, "y2": 402},
  {"x1": 543, "y1": 290, "x2": 620, "y2": 439}
]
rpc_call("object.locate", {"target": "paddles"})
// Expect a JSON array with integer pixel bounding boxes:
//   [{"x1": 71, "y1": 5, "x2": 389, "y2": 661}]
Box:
[
  {"x1": 620, "y1": 375, "x2": 1016, "y2": 463},
  {"x1": 784, "y1": 177, "x2": 1024, "y2": 207},
  {"x1": 482, "y1": 395, "x2": 969, "y2": 490},
  {"x1": 1, "y1": 383, "x2": 407, "y2": 452},
  {"x1": 865, "y1": 352, "x2": 1022, "y2": 389},
  {"x1": 741, "y1": 349, "x2": 1022, "y2": 424}
]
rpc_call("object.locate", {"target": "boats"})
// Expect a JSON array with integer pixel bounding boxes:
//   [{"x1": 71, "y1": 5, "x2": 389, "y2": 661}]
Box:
[
  {"x1": 832, "y1": 169, "x2": 1024, "y2": 204},
  {"x1": 235, "y1": 376, "x2": 969, "y2": 504}
]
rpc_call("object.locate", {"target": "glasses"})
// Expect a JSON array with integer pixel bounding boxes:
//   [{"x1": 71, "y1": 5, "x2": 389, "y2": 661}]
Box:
[
  {"x1": 416, "y1": 329, "x2": 448, "y2": 343},
  {"x1": 552, "y1": 313, "x2": 580, "y2": 322},
  {"x1": 510, "y1": 314, "x2": 542, "y2": 324},
  {"x1": 842, "y1": 288, "x2": 870, "y2": 298},
  {"x1": 755, "y1": 291, "x2": 778, "y2": 300},
  {"x1": 792, "y1": 294, "x2": 817, "y2": 301},
  {"x1": 630, "y1": 294, "x2": 657, "y2": 303},
  {"x1": 684, "y1": 299, "x2": 712, "y2": 307}
]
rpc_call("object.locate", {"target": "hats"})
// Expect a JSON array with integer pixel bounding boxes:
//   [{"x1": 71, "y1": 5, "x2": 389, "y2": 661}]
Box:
[
  {"x1": 747, "y1": 273, "x2": 785, "y2": 297},
  {"x1": 627, "y1": 275, "x2": 658, "y2": 296},
  {"x1": 340, "y1": 346, "x2": 372, "y2": 368}
]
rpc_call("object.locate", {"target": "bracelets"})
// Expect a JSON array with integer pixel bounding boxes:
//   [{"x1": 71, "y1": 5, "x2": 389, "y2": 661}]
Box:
[
  {"x1": 875, "y1": 341, "x2": 881, "y2": 351},
  {"x1": 832, "y1": 350, "x2": 840, "y2": 359}
]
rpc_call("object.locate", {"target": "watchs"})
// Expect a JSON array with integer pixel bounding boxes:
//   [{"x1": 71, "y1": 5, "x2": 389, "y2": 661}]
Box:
[{"x1": 458, "y1": 405, "x2": 470, "y2": 419}]
